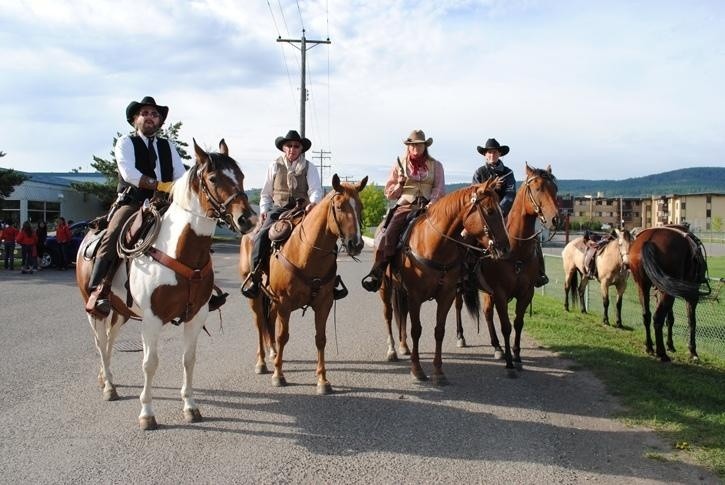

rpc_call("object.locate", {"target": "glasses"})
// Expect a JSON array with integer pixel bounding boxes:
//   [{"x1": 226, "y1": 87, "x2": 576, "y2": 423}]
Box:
[
  {"x1": 138, "y1": 111, "x2": 160, "y2": 118},
  {"x1": 284, "y1": 144, "x2": 301, "y2": 149}
]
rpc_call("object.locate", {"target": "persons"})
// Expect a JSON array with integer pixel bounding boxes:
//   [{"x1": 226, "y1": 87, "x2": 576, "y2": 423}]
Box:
[
  {"x1": 241, "y1": 129, "x2": 348, "y2": 301},
  {"x1": 83, "y1": 96, "x2": 229, "y2": 315},
  {"x1": 361, "y1": 129, "x2": 445, "y2": 293},
  {"x1": 0, "y1": 217, "x2": 74, "y2": 274},
  {"x1": 470, "y1": 139, "x2": 517, "y2": 219}
]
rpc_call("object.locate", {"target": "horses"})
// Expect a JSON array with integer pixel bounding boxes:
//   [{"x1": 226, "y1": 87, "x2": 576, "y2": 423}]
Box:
[
  {"x1": 455, "y1": 160, "x2": 565, "y2": 381},
  {"x1": 627, "y1": 223, "x2": 707, "y2": 364},
  {"x1": 238, "y1": 173, "x2": 369, "y2": 396},
  {"x1": 75, "y1": 137, "x2": 259, "y2": 431},
  {"x1": 373, "y1": 175, "x2": 512, "y2": 389},
  {"x1": 560, "y1": 227, "x2": 641, "y2": 331}
]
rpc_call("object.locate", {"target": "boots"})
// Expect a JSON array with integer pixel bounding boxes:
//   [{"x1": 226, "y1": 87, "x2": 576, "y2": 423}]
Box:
[
  {"x1": 209, "y1": 293, "x2": 228, "y2": 304},
  {"x1": 87, "y1": 261, "x2": 111, "y2": 314},
  {"x1": 364, "y1": 250, "x2": 393, "y2": 292}
]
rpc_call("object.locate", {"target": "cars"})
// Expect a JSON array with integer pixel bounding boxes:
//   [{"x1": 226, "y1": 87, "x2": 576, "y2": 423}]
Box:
[{"x1": 36, "y1": 221, "x2": 92, "y2": 268}]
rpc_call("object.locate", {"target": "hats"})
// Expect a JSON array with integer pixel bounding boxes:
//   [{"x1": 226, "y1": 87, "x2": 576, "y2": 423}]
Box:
[
  {"x1": 404, "y1": 130, "x2": 433, "y2": 146},
  {"x1": 275, "y1": 131, "x2": 311, "y2": 153},
  {"x1": 477, "y1": 139, "x2": 509, "y2": 156},
  {"x1": 126, "y1": 97, "x2": 168, "y2": 128}
]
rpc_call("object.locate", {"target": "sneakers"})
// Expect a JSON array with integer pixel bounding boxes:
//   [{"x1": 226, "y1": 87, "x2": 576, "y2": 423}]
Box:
[
  {"x1": 244, "y1": 281, "x2": 261, "y2": 297},
  {"x1": 334, "y1": 288, "x2": 348, "y2": 300},
  {"x1": 535, "y1": 277, "x2": 548, "y2": 287}
]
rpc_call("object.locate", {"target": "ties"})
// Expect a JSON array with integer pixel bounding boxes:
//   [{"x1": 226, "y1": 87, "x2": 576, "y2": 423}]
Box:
[{"x1": 148, "y1": 136, "x2": 157, "y2": 169}]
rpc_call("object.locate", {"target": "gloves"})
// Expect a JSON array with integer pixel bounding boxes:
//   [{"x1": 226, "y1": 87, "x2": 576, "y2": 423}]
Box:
[{"x1": 158, "y1": 181, "x2": 175, "y2": 192}]
